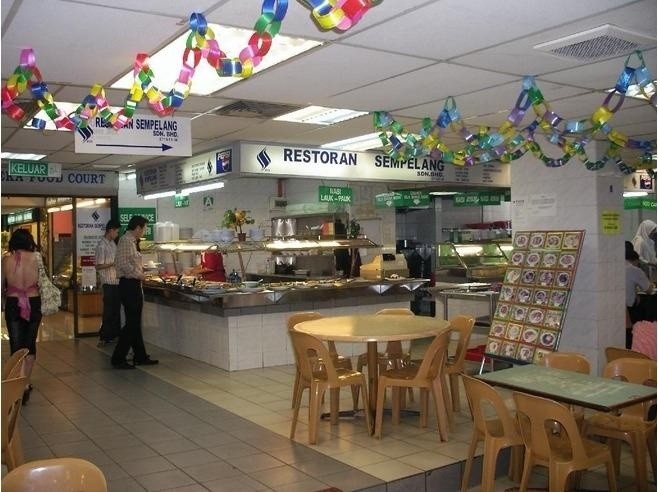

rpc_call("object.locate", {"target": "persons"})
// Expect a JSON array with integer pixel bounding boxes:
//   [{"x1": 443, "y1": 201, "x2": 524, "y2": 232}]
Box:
[
  {"x1": 630, "y1": 220, "x2": 655, "y2": 268},
  {"x1": 631, "y1": 301, "x2": 656, "y2": 360},
  {"x1": 110, "y1": 215, "x2": 158, "y2": 369},
  {"x1": 625, "y1": 240, "x2": 654, "y2": 348},
  {"x1": 189, "y1": 252, "x2": 226, "y2": 281},
  {"x1": 625, "y1": 253, "x2": 640, "y2": 267},
  {"x1": 94, "y1": 220, "x2": 119, "y2": 348},
  {"x1": 1, "y1": 228, "x2": 43, "y2": 406}
]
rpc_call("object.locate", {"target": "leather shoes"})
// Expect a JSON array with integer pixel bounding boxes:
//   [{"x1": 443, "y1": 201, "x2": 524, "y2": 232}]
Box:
[
  {"x1": 135, "y1": 358, "x2": 159, "y2": 367},
  {"x1": 113, "y1": 361, "x2": 133, "y2": 370}
]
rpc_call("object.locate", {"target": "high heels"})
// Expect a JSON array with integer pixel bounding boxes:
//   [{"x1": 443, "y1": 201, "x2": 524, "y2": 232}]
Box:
[{"x1": 22, "y1": 385, "x2": 31, "y2": 403}]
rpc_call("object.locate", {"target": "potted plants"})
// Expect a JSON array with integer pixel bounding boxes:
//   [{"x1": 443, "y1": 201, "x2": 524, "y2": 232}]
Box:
[{"x1": 220, "y1": 208, "x2": 255, "y2": 242}]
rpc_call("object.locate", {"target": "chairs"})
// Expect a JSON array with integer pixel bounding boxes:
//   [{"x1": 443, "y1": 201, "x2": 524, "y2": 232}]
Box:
[
  {"x1": 1, "y1": 376, "x2": 25, "y2": 472},
  {"x1": 512, "y1": 391, "x2": 617, "y2": 492},
  {"x1": 1, "y1": 458, "x2": 108, "y2": 492},
  {"x1": 353, "y1": 308, "x2": 416, "y2": 409},
  {"x1": 375, "y1": 323, "x2": 457, "y2": 443},
  {"x1": 288, "y1": 329, "x2": 375, "y2": 445},
  {"x1": 1, "y1": 348, "x2": 29, "y2": 380},
  {"x1": 581, "y1": 358, "x2": 657, "y2": 492},
  {"x1": 605, "y1": 346, "x2": 651, "y2": 363},
  {"x1": 287, "y1": 312, "x2": 356, "y2": 410},
  {"x1": 410, "y1": 314, "x2": 475, "y2": 429},
  {"x1": 508, "y1": 353, "x2": 590, "y2": 481},
  {"x1": 461, "y1": 374, "x2": 525, "y2": 492}
]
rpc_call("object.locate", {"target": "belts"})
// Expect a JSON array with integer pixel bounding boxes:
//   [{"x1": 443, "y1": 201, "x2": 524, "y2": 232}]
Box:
[{"x1": 118, "y1": 277, "x2": 140, "y2": 285}]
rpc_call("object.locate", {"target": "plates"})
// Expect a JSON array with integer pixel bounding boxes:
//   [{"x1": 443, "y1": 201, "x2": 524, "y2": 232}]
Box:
[
  {"x1": 319, "y1": 281, "x2": 336, "y2": 288},
  {"x1": 268, "y1": 284, "x2": 292, "y2": 290},
  {"x1": 333, "y1": 281, "x2": 348, "y2": 285},
  {"x1": 178, "y1": 228, "x2": 194, "y2": 241},
  {"x1": 295, "y1": 282, "x2": 311, "y2": 288},
  {"x1": 154, "y1": 221, "x2": 180, "y2": 240},
  {"x1": 238, "y1": 286, "x2": 262, "y2": 293}
]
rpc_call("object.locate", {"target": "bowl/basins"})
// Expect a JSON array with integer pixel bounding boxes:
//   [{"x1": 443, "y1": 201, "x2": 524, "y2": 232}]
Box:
[
  {"x1": 241, "y1": 281, "x2": 260, "y2": 287},
  {"x1": 249, "y1": 230, "x2": 266, "y2": 240},
  {"x1": 200, "y1": 230, "x2": 233, "y2": 242},
  {"x1": 81, "y1": 285, "x2": 99, "y2": 294}
]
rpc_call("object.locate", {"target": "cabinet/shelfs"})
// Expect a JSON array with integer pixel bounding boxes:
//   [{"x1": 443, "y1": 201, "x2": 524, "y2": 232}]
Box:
[
  {"x1": 438, "y1": 289, "x2": 500, "y2": 323},
  {"x1": 121, "y1": 235, "x2": 430, "y2": 373},
  {"x1": 435, "y1": 242, "x2": 514, "y2": 320},
  {"x1": 53, "y1": 265, "x2": 103, "y2": 315}
]
